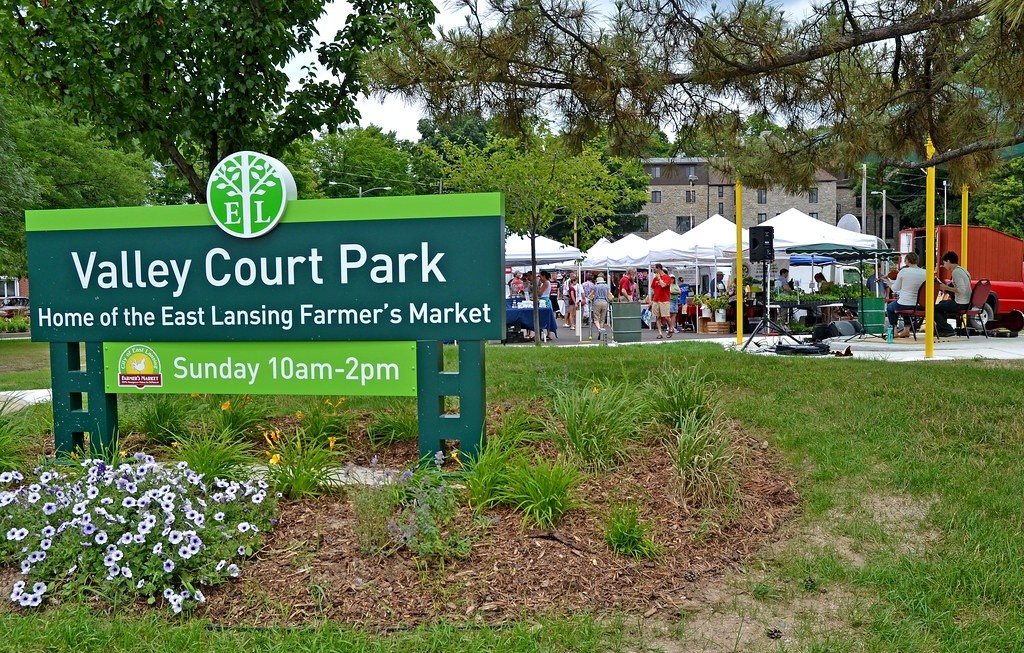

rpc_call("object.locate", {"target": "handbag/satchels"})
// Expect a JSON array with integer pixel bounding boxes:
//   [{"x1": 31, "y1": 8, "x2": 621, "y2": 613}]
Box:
[{"x1": 607, "y1": 289, "x2": 614, "y2": 301}]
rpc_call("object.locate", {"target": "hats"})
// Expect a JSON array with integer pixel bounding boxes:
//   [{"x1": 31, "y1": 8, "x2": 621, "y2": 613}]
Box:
[
  {"x1": 514, "y1": 271, "x2": 519, "y2": 278},
  {"x1": 557, "y1": 274, "x2": 564, "y2": 280},
  {"x1": 717, "y1": 271, "x2": 724, "y2": 275},
  {"x1": 597, "y1": 278, "x2": 606, "y2": 284}
]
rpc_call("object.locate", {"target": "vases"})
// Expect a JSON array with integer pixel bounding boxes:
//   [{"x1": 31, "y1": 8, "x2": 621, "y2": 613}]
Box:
[
  {"x1": 701, "y1": 303, "x2": 712, "y2": 317},
  {"x1": 714, "y1": 308, "x2": 726, "y2": 322}
]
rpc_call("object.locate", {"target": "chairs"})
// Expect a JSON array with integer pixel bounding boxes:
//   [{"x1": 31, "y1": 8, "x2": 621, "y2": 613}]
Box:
[
  {"x1": 892, "y1": 277, "x2": 941, "y2": 340},
  {"x1": 951, "y1": 277, "x2": 992, "y2": 338}
]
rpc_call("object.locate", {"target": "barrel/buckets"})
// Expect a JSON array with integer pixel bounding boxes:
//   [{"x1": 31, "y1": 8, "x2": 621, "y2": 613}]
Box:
[
  {"x1": 857, "y1": 297, "x2": 885, "y2": 334},
  {"x1": 702, "y1": 304, "x2": 711, "y2": 317},
  {"x1": 715, "y1": 309, "x2": 726, "y2": 322},
  {"x1": 612, "y1": 301, "x2": 641, "y2": 343}
]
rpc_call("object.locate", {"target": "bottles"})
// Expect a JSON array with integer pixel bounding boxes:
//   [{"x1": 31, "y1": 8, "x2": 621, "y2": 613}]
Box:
[{"x1": 887, "y1": 325, "x2": 893, "y2": 345}]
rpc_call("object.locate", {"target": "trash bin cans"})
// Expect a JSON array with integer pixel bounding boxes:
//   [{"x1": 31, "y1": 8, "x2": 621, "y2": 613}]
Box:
[
  {"x1": 612, "y1": 301, "x2": 641, "y2": 342},
  {"x1": 858, "y1": 297, "x2": 886, "y2": 334}
]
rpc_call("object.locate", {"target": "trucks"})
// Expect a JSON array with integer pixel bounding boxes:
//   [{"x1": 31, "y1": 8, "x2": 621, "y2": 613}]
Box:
[
  {"x1": 787, "y1": 265, "x2": 868, "y2": 294},
  {"x1": 897, "y1": 224, "x2": 1024, "y2": 332}
]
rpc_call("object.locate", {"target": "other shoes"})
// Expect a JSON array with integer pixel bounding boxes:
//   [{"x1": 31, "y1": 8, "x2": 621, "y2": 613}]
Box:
[
  {"x1": 563, "y1": 324, "x2": 569, "y2": 328},
  {"x1": 657, "y1": 335, "x2": 661, "y2": 339},
  {"x1": 570, "y1": 327, "x2": 575, "y2": 329},
  {"x1": 885, "y1": 333, "x2": 899, "y2": 339},
  {"x1": 899, "y1": 332, "x2": 911, "y2": 338},
  {"x1": 937, "y1": 330, "x2": 955, "y2": 338},
  {"x1": 672, "y1": 328, "x2": 679, "y2": 333},
  {"x1": 666, "y1": 330, "x2": 673, "y2": 338}
]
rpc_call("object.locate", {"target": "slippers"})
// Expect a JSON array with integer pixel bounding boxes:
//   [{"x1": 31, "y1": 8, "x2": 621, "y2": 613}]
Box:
[{"x1": 542, "y1": 336, "x2": 553, "y2": 341}]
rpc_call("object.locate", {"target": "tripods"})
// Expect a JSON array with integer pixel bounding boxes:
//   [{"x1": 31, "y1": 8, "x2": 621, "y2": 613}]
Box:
[
  {"x1": 844, "y1": 248, "x2": 882, "y2": 344},
  {"x1": 741, "y1": 261, "x2": 803, "y2": 352}
]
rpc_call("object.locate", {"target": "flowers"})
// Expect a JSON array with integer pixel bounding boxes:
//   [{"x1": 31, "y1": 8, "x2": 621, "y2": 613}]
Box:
[{"x1": 693, "y1": 293, "x2": 731, "y2": 312}]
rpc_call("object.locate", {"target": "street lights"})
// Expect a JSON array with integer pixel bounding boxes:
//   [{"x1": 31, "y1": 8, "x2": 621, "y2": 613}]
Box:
[
  {"x1": 871, "y1": 190, "x2": 886, "y2": 275},
  {"x1": 329, "y1": 181, "x2": 391, "y2": 198}
]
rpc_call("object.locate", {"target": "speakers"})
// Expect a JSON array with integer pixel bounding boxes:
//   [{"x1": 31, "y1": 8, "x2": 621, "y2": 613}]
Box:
[{"x1": 749, "y1": 226, "x2": 775, "y2": 264}]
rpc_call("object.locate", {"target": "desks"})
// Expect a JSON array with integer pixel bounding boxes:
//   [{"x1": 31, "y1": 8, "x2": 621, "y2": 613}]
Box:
[
  {"x1": 765, "y1": 298, "x2": 859, "y2": 327},
  {"x1": 677, "y1": 304, "x2": 735, "y2": 332},
  {"x1": 504, "y1": 307, "x2": 558, "y2": 343}
]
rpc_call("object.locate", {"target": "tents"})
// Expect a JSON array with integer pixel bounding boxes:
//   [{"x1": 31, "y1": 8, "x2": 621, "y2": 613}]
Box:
[
  {"x1": 506, "y1": 228, "x2": 647, "y2": 301},
  {"x1": 647, "y1": 207, "x2": 878, "y2": 303}
]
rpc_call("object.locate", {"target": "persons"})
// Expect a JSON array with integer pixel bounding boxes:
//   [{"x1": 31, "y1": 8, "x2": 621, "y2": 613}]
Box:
[
  {"x1": 563, "y1": 263, "x2": 693, "y2": 339},
  {"x1": 711, "y1": 268, "x2": 827, "y2": 323},
  {"x1": 507, "y1": 270, "x2": 560, "y2": 339},
  {"x1": 866, "y1": 251, "x2": 972, "y2": 338}
]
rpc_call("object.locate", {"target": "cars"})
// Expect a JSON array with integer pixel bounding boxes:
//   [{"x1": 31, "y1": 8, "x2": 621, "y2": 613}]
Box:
[{"x1": 0, "y1": 297, "x2": 30, "y2": 318}]
